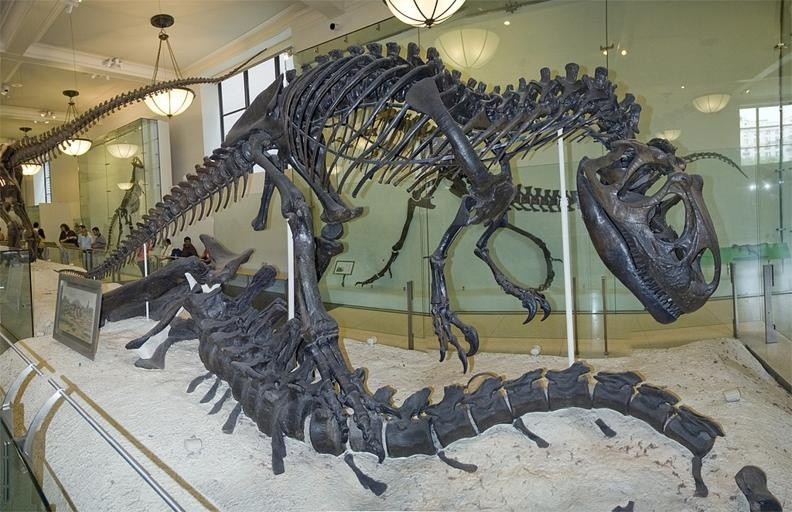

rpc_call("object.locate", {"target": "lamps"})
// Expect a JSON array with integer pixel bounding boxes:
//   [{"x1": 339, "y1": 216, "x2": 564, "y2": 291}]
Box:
[
  {"x1": 57, "y1": 90, "x2": 92, "y2": 156},
  {"x1": 654, "y1": 129, "x2": 681, "y2": 141},
  {"x1": 434, "y1": 29, "x2": 499, "y2": 69},
  {"x1": 19, "y1": 127, "x2": 41, "y2": 176},
  {"x1": 691, "y1": 95, "x2": 731, "y2": 113},
  {"x1": 143, "y1": 14, "x2": 195, "y2": 118},
  {"x1": 118, "y1": 183, "x2": 133, "y2": 189},
  {"x1": 384, "y1": 0, "x2": 468, "y2": 28},
  {"x1": 108, "y1": 144, "x2": 138, "y2": 158}
]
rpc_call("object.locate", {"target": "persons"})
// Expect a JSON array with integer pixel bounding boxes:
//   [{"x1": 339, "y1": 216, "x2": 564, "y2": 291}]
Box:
[
  {"x1": 181, "y1": 236, "x2": 197, "y2": 256},
  {"x1": 33, "y1": 222, "x2": 40, "y2": 235},
  {"x1": 78, "y1": 224, "x2": 94, "y2": 272},
  {"x1": 59, "y1": 222, "x2": 79, "y2": 266},
  {"x1": 38, "y1": 228, "x2": 51, "y2": 259},
  {"x1": 92, "y1": 226, "x2": 106, "y2": 268},
  {"x1": 201, "y1": 247, "x2": 210, "y2": 261},
  {"x1": 136, "y1": 239, "x2": 152, "y2": 276},
  {"x1": 159, "y1": 238, "x2": 175, "y2": 268}
]
